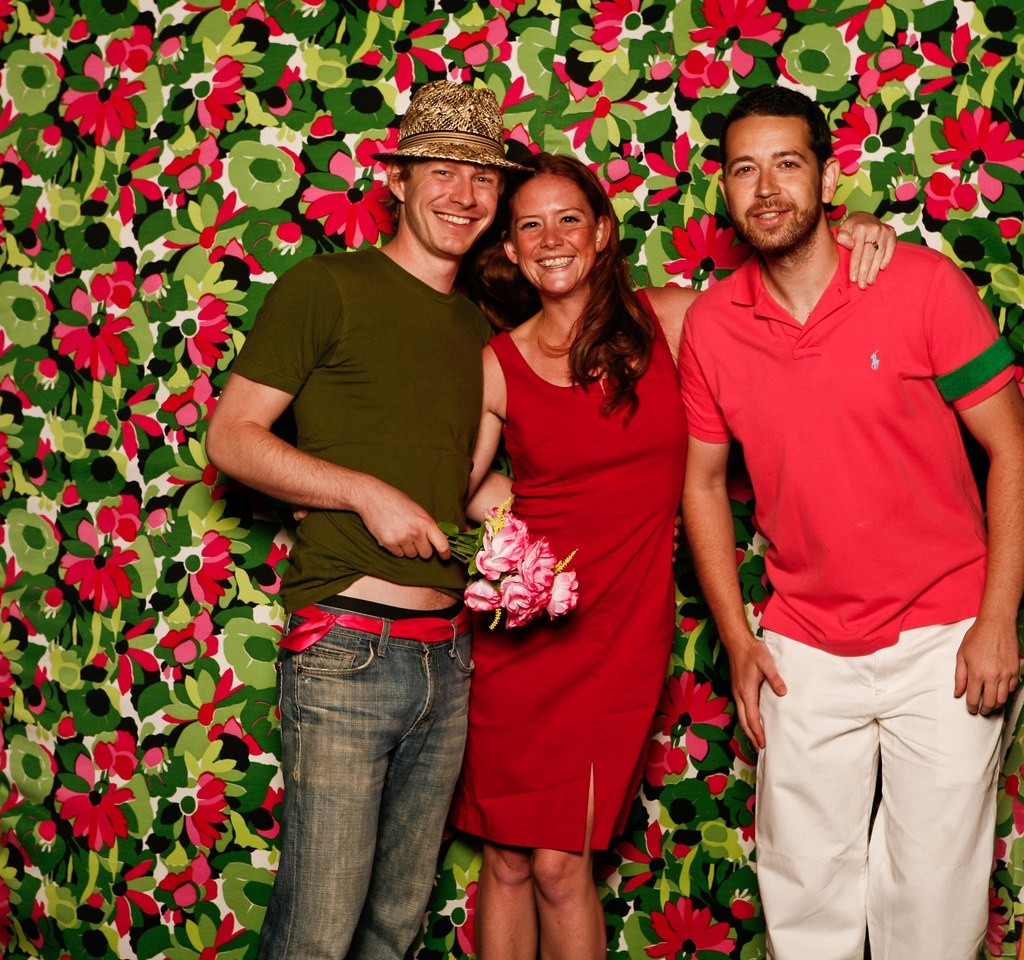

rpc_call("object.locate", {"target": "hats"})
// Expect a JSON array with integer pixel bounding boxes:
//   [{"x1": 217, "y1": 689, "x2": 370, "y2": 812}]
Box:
[{"x1": 368, "y1": 80, "x2": 536, "y2": 173}]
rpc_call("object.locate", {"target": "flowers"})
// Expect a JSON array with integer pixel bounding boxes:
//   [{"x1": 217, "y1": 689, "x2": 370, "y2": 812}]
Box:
[{"x1": 436, "y1": 496, "x2": 581, "y2": 632}]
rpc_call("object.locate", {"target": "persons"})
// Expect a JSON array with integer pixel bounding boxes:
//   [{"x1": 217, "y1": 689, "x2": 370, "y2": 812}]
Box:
[
  {"x1": 679, "y1": 85, "x2": 1024, "y2": 960},
  {"x1": 457, "y1": 153, "x2": 897, "y2": 960},
  {"x1": 202, "y1": 85, "x2": 530, "y2": 960}
]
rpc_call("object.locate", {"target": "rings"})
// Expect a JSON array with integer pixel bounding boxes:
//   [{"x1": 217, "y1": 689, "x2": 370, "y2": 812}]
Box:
[{"x1": 864, "y1": 240, "x2": 878, "y2": 250}]
